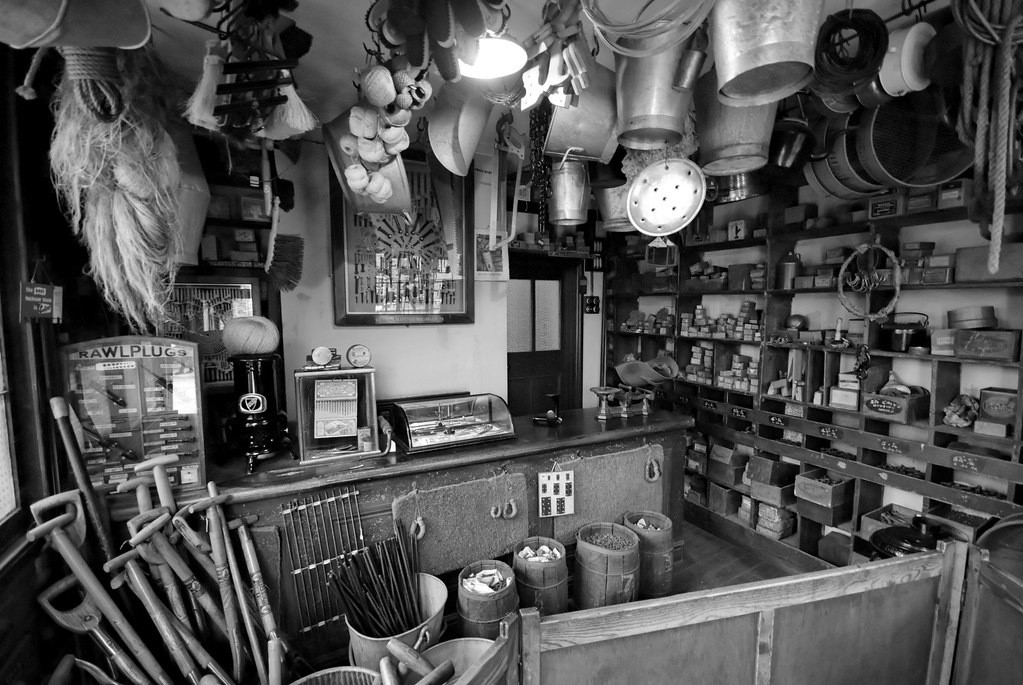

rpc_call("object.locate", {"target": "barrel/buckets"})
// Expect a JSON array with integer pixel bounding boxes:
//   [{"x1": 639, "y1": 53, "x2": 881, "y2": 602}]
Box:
[
  {"x1": 456, "y1": 559, "x2": 520, "y2": 641},
  {"x1": 612, "y1": 0, "x2": 710, "y2": 150},
  {"x1": 708, "y1": 0, "x2": 826, "y2": 108},
  {"x1": 544, "y1": 161, "x2": 591, "y2": 226},
  {"x1": 511, "y1": 535, "x2": 570, "y2": 616},
  {"x1": 574, "y1": 521, "x2": 641, "y2": 610},
  {"x1": 344, "y1": 571, "x2": 449, "y2": 674},
  {"x1": 397, "y1": 637, "x2": 495, "y2": 685},
  {"x1": 691, "y1": 61, "x2": 779, "y2": 176},
  {"x1": 540, "y1": 61, "x2": 619, "y2": 165},
  {"x1": 623, "y1": 509, "x2": 675, "y2": 596},
  {"x1": 287, "y1": 666, "x2": 383, "y2": 685}
]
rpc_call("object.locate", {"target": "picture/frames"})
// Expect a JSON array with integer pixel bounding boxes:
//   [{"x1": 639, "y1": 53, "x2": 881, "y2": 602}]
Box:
[
  {"x1": 329, "y1": 145, "x2": 475, "y2": 327},
  {"x1": 154, "y1": 277, "x2": 261, "y2": 395},
  {"x1": 475, "y1": 227, "x2": 509, "y2": 282}
]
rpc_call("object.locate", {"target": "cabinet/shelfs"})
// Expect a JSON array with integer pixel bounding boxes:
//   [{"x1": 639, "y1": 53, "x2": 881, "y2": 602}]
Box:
[
  {"x1": 599, "y1": 168, "x2": 1023, "y2": 572},
  {"x1": 115, "y1": 131, "x2": 288, "y2": 463}
]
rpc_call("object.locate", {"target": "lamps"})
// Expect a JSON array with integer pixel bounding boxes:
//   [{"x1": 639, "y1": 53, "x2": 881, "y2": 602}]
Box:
[{"x1": 457, "y1": 27, "x2": 529, "y2": 79}]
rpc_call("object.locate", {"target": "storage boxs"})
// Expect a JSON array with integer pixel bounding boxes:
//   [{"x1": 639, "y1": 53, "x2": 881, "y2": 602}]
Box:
[
  {"x1": 207, "y1": 189, "x2": 270, "y2": 262},
  {"x1": 619, "y1": 241, "x2": 1023, "y2": 439},
  {"x1": 685, "y1": 438, "x2": 995, "y2": 568},
  {"x1": 710, "y1": 177, "x2": 974, "y2": 242},
  {"x1": 511, "y1": 230, "x2": 589, "y2": 256}
]
